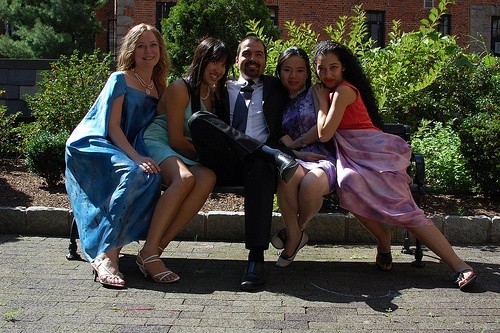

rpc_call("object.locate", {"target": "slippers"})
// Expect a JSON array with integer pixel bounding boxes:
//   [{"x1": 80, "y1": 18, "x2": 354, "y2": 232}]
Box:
[
  {"x1": 455, "y1": 269, "x2": 477, "y2": 290},
  {"x1": 376, "y1": 252, "x2": 393, "y2": 271}
]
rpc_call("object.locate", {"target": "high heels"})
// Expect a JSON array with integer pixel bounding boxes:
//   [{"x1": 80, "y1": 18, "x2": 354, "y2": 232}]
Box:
[
  {"x1": 136, "y1": 246, "x2": 180, "y2": 283},
  {"x1": 90, "y1": 256, "x2": 124, "y2": 287}
]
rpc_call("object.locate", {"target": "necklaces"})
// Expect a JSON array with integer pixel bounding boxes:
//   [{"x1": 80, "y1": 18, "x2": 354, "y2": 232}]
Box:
[
  {"x1": 201, "y1": 86, "x2": 210, "y2": 100},
  {"x1": 132, "y1": 68, "x2": 156, "y2": 95}
]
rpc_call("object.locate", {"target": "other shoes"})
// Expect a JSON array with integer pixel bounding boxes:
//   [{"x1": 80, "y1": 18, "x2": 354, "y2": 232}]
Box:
[
  {"x1": 276, "y1": 232, "x2": 308, "y2": 267},
  {"x1": 271, "y1": 228, "x2": 285, "y2": 249}
]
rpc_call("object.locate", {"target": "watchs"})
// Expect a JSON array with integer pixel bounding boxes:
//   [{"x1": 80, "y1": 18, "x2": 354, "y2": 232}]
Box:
[{"x1": 301, "y1": 133, "x2": 306, "y2": 148}]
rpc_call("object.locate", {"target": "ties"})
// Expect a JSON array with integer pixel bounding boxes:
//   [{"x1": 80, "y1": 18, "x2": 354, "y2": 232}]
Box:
[{"x1": 232, "y1": 80, "x2": 257, "y2": 135}]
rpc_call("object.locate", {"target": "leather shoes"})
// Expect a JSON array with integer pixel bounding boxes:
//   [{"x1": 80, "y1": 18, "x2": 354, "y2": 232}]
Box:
[
  {"x1": 276, "y1": 153, "x2": 300, "y2": 185},
  {"x1": 241, "y1": 265, "x2": 271, "y2": 286}
]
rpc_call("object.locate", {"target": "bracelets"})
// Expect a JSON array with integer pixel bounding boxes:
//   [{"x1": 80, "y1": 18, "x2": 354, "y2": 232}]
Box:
[{"x1": 134, "y1": 155, "x2": 143, "y2": 163}]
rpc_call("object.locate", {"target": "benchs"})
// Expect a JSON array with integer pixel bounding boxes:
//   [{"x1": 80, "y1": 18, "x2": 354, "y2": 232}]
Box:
[{"x1": 65, "y1": 123, "x2": 431, "y2": 269}]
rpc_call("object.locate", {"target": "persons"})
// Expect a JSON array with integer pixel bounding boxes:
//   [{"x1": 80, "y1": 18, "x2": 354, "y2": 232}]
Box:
[
  {"x1": 311, "y1": 40, "x2": 478, "y2": 291},
  {"x1": 136, "y1": 36, "x2": 338, "y2": 291},
  {"x1": 65, "y1": 22, "x2": 171, "y2": 287}
]
